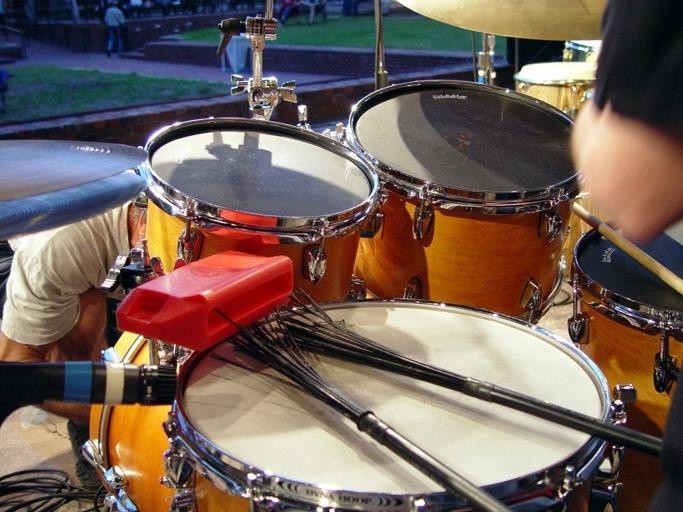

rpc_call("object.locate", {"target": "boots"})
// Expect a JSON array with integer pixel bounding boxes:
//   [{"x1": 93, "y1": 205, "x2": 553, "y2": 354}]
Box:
[{"x1": 67, "y1": 421, "x2": 101, "y2": 486}]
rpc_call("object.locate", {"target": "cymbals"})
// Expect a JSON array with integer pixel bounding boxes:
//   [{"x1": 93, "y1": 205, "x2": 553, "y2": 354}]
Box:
[{"x1": 394, "y1": 0, "x2": 608, "y2": 40}]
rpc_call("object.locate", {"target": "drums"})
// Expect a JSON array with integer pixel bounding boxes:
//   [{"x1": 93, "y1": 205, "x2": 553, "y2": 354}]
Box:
[
  {"x1": 84, "y1": 330, "x2": 174, "y2": 510},
  {"x1": 514, "y1": 60, "x2": 597, "y2": 122},
  {"x1": 146, "y1": 116, "x2": 381, "y2": 314},
  {"x1": 563, "y1": 40, "x2": 602, "y2": 62},
  {"x1": 346, "y1": 80, "x2": 583, "y2": 324},
  {"x1": 567, "y1": 218, "x2": 683, "y2": 512},
  {"x1": 164, "y1": 297, "x2": 625, "y2": 511}
]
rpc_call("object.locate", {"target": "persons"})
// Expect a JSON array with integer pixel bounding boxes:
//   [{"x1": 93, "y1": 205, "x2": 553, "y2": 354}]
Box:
[
  {"x1": 276, "y1": 0, "x2": 302, "y2": 29},
  {"x1": 103, "y1": 1, "x2": 127, "y2": 58},
  {"x1": 0, "y1": 194, "x2": 147, "y2": 489},
  {"x1": 569, "y1": 0, "x2": 683, "y2": 512}
]
